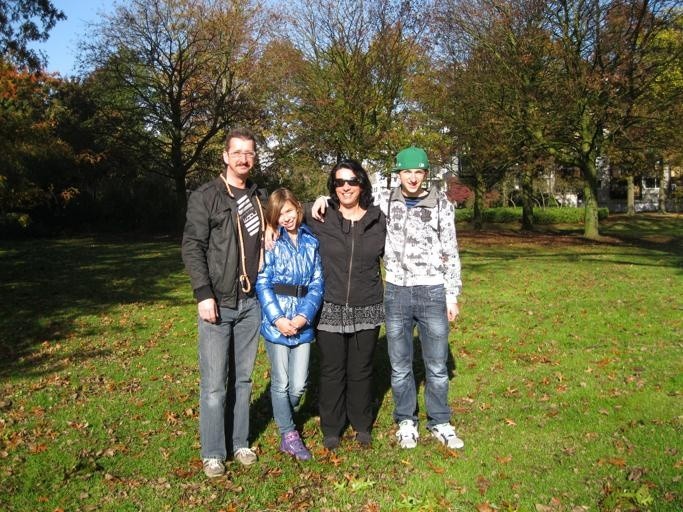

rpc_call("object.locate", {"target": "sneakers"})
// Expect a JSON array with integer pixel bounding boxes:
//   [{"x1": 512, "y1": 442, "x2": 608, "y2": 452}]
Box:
[
  {"x1": 356, "y1": 432, "x2": 371, "y2": 446},
  {"x1": 431, "y1": 422, "x2": 464, "y2": 448},
  {"x1": 232, "y1": 448, "x2": 257, "y2": 465},
  {"x1": 203, "y1": 458, "x2": 225, "y2": 479},
  {"x1": 280, "y1": 430, "x2": 312, "y2": 461},
  {"x1": 395, "y1": 419, "x2": 418, "y2": 449},
  {"x1": 323, "y1": 436, "x2": 340, "y2": 452}
]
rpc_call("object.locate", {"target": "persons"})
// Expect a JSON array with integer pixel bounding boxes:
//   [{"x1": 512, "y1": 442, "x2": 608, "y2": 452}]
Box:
[
  {"x1": 264, "y1": 158, "x2": 385, "y2": 449},
  {"x1": 310, "y1": 145, "x2": 465, "y2": 450},
  {"x1": 179, "y1": 127, "x2": 267, "y2": 480},
  {"x1": 254, "y1": 187, "x2": 324, "y2": 461}
]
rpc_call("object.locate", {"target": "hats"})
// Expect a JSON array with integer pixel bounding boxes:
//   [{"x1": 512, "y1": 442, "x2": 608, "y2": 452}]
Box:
[{"x1": 391, "y1": 147, "x2": 429, "y2": 173}]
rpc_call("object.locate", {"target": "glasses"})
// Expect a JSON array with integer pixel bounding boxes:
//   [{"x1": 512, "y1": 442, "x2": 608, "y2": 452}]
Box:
[{"x1": 334, "y1": 177, "x2": 359, "y2": 187}]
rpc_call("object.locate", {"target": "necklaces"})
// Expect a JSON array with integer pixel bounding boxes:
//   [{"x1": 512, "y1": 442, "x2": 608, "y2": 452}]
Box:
[{"x1": 220, "y1": 171, "x2": 264, "y2": 293}]
